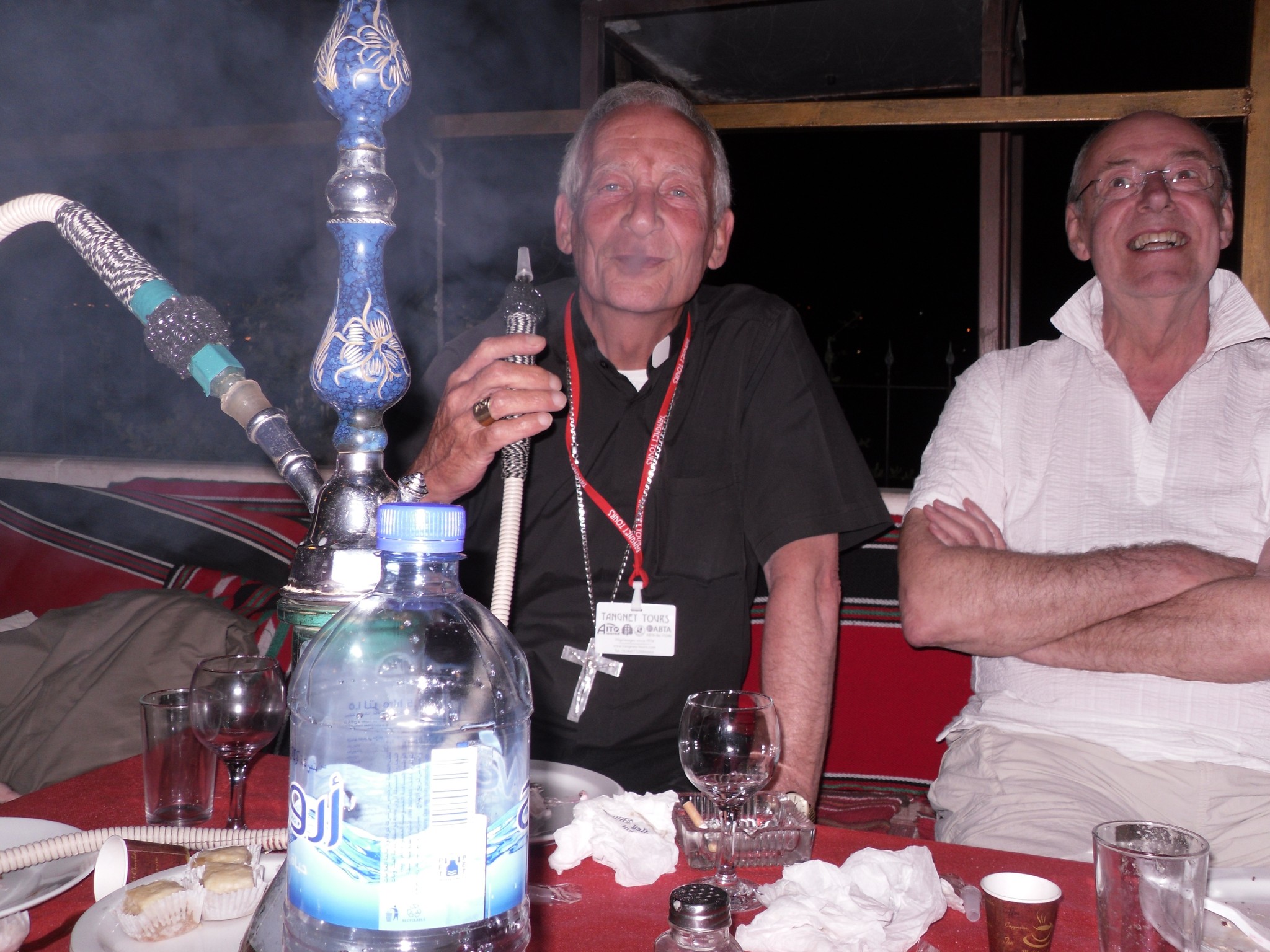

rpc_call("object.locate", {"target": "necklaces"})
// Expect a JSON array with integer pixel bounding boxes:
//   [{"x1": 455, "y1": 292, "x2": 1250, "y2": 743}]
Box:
[{"x1": 560, "y1": 356, "x2": 678, "y2": 723}]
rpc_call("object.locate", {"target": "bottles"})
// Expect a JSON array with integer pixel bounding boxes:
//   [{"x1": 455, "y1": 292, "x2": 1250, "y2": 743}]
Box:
[
  {"x1": 283, "y1": 502, "x2": 535, "y2": 951},
  {"x1": 652, "y1": 881, "x2": 747, "y2": 952}
]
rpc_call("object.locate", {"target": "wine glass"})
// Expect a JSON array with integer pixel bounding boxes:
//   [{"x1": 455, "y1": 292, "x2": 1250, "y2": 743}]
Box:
[
  {"x1": 677, "y1": 689, "x2": 783, "y2": 914},
  {"x1": 187, "y1": 654, "x2": 289, "y2": 829}
]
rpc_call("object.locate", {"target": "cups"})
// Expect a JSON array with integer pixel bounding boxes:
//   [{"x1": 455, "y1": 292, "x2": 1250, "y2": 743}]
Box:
[
  {"x1": 1088, "y1": 820, "x2": 1210, "y2": 952},
  {"x1": 980, "y1": 872, "x2": 1062, "y2": 952},
  {"x1": 137, "y1": 688, "x2": 217, "y2": 827},
  {"x1": 93, "y1": 834, "x2": 191, "y2": 904}
]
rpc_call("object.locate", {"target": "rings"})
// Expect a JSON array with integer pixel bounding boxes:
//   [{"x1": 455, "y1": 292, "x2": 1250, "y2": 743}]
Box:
[{"x1": 472, "y1": 397, "x2": 495, "y2": 426}]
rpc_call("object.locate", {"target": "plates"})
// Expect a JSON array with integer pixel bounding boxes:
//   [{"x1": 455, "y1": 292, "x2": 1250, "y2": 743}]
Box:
[
  {"x1": 0, "y1": 815, "x2": 99, "y2": 919},
  {"x1": 1137, "y1": 864, "x2": 1270, "y2": 952},
  {"x1": 528, "y1": 759, "x2": 625, "y2": 845},
  {"x1": 69, "y1": 853, "x2": 288, "y2": 951}
]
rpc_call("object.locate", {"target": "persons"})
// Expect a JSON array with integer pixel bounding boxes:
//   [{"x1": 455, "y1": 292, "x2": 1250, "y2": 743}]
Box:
[
  {"x1": 371, "y1": 79, "x2": 898, "y2": 824},
  {"x1": 900, "y1": 111, "x2": 1270, "y2": 882}
]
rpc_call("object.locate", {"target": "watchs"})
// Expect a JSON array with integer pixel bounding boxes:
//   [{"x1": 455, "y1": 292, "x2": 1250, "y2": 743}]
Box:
[{"x1": 785, "y1": 792, "x2": 815, "y2": 824}]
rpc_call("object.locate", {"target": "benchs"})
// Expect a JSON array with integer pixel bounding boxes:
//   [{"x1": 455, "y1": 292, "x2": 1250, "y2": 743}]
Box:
[{"x1": 0, "y1": 472, "x2": 981, "y2": 843}]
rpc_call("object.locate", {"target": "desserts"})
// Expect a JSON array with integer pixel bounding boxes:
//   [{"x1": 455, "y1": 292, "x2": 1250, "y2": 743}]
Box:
[{"x1": 117, "y1": 843, "x2": 270, "y2": 942}]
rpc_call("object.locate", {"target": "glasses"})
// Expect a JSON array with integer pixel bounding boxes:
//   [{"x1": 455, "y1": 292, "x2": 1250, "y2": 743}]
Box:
[{"x1": 1074, "y1": 158, "x2": 1224, "y2": 200}]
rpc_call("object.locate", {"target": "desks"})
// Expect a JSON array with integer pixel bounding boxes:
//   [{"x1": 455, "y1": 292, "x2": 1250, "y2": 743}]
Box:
[{"x1": 0, "y1": 727, "x2": 1270, "y2": 952}]
